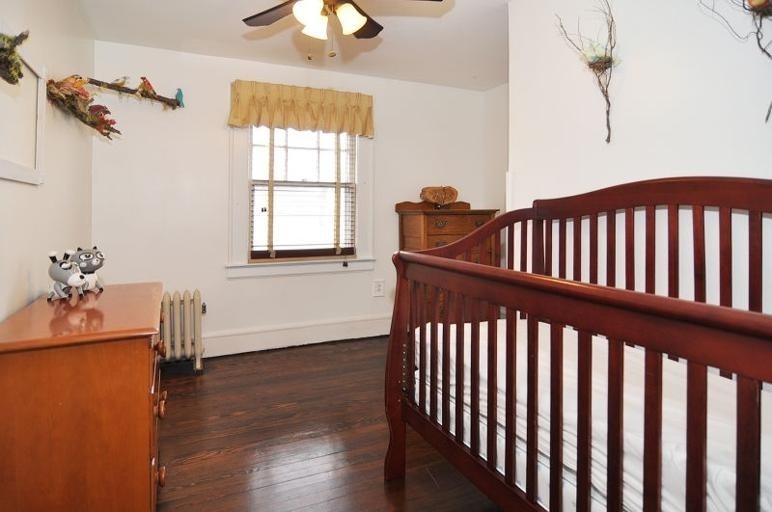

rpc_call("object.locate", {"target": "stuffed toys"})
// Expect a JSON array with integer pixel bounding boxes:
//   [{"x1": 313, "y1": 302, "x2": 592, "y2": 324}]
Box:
[
  {"x1": 71, "y1": 245, "x2": 108, "y2": 295},
  {"x1": 45, "y1": 249, "x2": 87, "y2": 303}
]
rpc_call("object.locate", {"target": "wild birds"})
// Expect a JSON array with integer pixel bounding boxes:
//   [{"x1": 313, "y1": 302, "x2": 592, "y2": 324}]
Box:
[
  {"x1": 140, "y1": 76, "x2": 158, "y2": 97},
  {"x1": 175, "y1": 88, "x2": 185, "y2": 109},
  {"x1": 109, "y1": 75, "x2": 128, "y2": 88}
]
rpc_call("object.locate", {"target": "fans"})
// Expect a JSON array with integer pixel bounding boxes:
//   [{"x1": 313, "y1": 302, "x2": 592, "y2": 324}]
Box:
[{"x1": 240, "y1": 0, "x2": 443, "y2": 40}]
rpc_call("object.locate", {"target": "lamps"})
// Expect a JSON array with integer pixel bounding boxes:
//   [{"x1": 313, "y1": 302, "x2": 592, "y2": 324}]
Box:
[{"x1": 292, "y1": 1, "x2": 369, "y2": 59}]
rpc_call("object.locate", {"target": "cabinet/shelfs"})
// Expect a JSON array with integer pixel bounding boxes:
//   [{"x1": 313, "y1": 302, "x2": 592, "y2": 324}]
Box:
[
  {"x1": 395, "y1": 200, "x2": 501, "y2": 328},
  {"x1": 0, "y1": 280, "x2": 172, "y2": 512}
]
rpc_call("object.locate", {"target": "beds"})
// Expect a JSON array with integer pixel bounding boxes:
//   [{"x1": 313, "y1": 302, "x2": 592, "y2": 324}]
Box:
[{"x1": 380, "y1": 171, "x2": 772, "y2": 511}]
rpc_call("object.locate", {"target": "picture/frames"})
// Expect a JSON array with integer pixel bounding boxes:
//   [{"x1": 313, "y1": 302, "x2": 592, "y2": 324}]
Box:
[{"x1": 1, "y1": 45, "x2": 46, "y2": 189}]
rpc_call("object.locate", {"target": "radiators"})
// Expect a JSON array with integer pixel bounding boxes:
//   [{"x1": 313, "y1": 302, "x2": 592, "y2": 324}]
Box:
[{"x1": 157, "y1": 289, "x2": 204, "y2": 373}]
